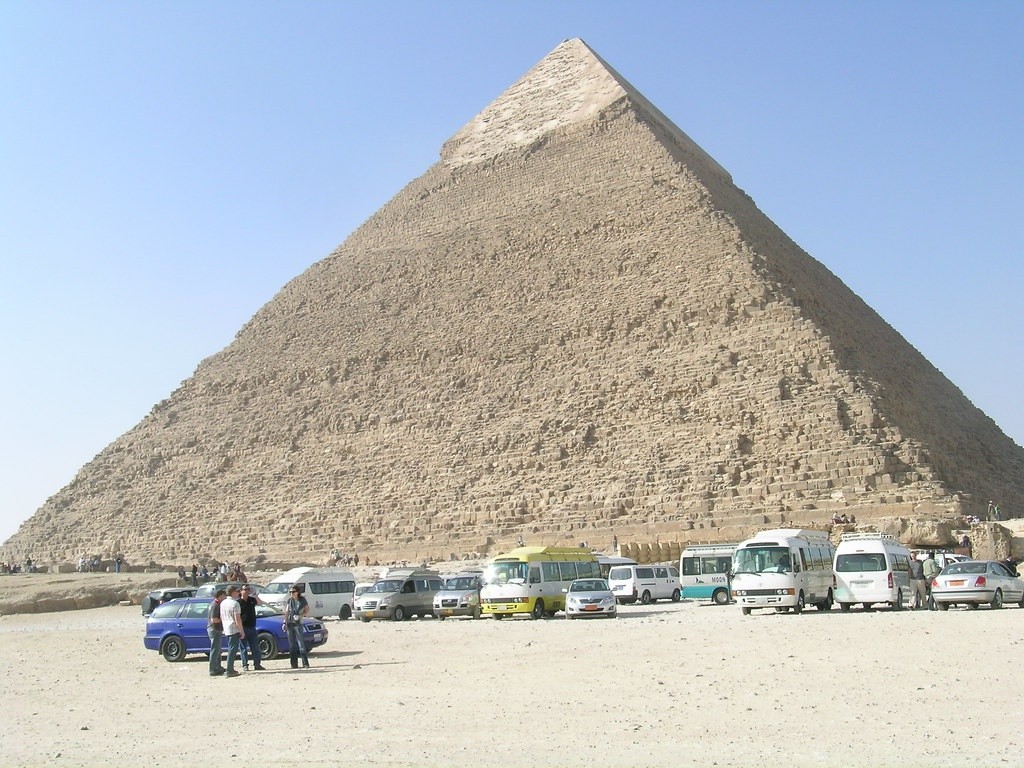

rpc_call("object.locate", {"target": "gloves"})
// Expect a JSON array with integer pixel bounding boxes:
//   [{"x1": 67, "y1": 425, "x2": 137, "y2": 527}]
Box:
[
  {"x1": 282, "y1": 620, "x2": 288, "y2": 633},
  {"x1": 293, "y1": 615, "x2": 300, "y2": 621}
]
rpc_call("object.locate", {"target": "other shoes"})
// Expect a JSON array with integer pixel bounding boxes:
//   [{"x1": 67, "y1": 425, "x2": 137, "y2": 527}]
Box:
[
  {"x1": 291, "y1": 667, "x2": 297, "y2": 669},
  {"x1": 233, "y1": 670, "x2": 239, "y2": 676},
  {"x1": 243, "y1": 666, "x2": 248, "y2": 670},
  {"x1": 255, "y1": 665, "x2": 266, "y2": 670},
  {"x1": 303, "y1": 664, "x2": 309, "y2": 669}
]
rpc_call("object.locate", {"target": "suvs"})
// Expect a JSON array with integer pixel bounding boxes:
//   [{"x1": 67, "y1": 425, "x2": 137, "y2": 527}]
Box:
[
  {"x1": 141, "y1": 588, "x2": 198, "y2": 619},
  {"x1": 143, "y1": 596, "x2": 329, "y2": 662}
]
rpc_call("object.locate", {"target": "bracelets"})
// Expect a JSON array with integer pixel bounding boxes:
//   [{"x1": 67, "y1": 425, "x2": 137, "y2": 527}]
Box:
[
  {"x1": 282, "y1": 620, "x2": 287, "y2": 624},
  {"x1": 252, "y1": 593, "x2": 256, "y2": 596}
]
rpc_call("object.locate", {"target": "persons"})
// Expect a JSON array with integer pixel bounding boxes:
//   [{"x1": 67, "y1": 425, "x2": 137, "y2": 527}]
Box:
[
  {"x1": 517, "y1": 536, "x2": 524, "y2": 547},
  {"x1": 958, "y1": 500, "x2": 1017, "y2": 574},
  {"x1": 922, "y1": 552, "x2": 942, "y2": 611},
  {"x1": 578, "y1": 540, "x2": 588, "y2": 548},
  {"x1": 613, "y1": 535, "x2": 618, "y2": 553},
  {"x1": 178, "y1": 561, "x2": 247, "y2": 583},
  {"x1": 346, "y1": 553, "x2": 430, "y2": 567},
  {"x1": 907, "y1": 552, "x2": 929, "y2": 610},
  {"x1": 206, "y1": 583, "x2": 267, "y2": 676},
  {"x1": 2, "y1": 556, "x2": 122, "y2": 573},
  {"x1": 281, "y1": 585, "x2": 310, "y2": 669},
  {"x1": 833, "y1": 511, "x2": 856, "y2": 524}
]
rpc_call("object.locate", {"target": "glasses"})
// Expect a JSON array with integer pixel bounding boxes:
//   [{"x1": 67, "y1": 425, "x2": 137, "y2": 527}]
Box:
[
  {"x1": 244, "y1": 589, "x2": 250, "y2": 591},
  {"x1": 289, "y1": 591, "x2": 297, "y2": 593}
]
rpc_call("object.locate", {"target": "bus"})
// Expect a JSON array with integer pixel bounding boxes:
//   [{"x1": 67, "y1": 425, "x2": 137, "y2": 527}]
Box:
[
  {"x1": 593, "y1": 553, "x2": 639, "y2": 585},
  {"x1": 730, "y1": 527, "x2": 837, "y2": 615},
  {"x1": 680, "y1": 541, "x2": 745, "y2": 606},
  {"x1": 831, "y1": 533, "x2": 917, "y2": 613},
  {"x1": 479, "y1": 546, "x2": 601, "y2": 620}
]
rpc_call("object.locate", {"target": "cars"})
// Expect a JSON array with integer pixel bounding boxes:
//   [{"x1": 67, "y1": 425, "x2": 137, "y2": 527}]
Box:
[
  {"x1": 561, "y1": 577, "x2": 618, "y2": 620},
  {"x1": 930, "y1": 559, "x2": 1024, "y2": 609}
]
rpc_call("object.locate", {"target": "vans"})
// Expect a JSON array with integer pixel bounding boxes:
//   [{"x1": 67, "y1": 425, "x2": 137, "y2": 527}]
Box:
[
  {"x1": 431, "y1": 570, "x2": 484, "y2": 620},
  {"x1": 353, "y1": 582, "x2": 375, "y2": 608},
  {"x1": 356, "y1": 569, "x2": 447, "y2": 622},
  {"x1": 909, "y1": 546, "x2": 977, "y2": 578},
  {"x1": 255, "y1": 565, "x2": 358, "y2": 621},
  {"x1": 194, "y1": 584, "x2": 272, "y2": 617},
  {"x1": 607, "y1": 565, "x2": 682, "y2": 605}
]
